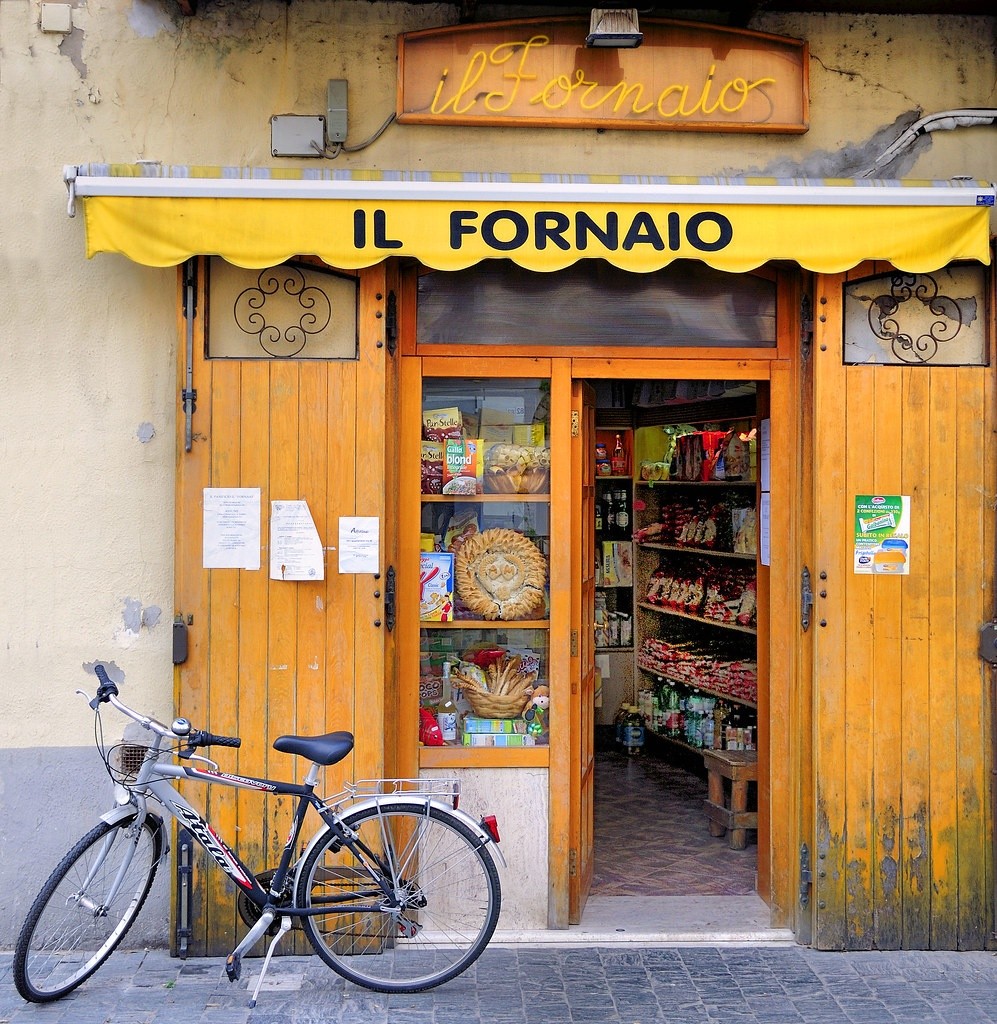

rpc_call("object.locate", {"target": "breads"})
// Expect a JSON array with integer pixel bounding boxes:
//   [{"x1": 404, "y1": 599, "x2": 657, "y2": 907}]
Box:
[
  {"x1": 455, "y1": 654, "x2": 537, "y2": 697},
  {"x1": 456, "y1": 526, "x2": 548, "y2": 620}
]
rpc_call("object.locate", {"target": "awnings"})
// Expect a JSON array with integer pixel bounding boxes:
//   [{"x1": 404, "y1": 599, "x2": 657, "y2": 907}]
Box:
[{"x1": 64, "y1": 163, "x2": 996, "y2": 276}]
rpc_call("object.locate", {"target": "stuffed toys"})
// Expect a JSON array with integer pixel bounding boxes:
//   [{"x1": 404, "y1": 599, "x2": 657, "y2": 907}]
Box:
[{"x1": 522, "y1": 681, "x2": 550, "y2": 745}]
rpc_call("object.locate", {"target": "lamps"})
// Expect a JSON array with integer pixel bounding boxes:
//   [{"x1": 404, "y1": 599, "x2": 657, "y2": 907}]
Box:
[{"x1": 585, "y1": 7, "x2": 643, "y2": 48}]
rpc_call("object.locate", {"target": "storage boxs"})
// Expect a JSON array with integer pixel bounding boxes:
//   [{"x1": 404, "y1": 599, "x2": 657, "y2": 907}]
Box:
[
  {"x1": 460, "y1": 715, "x2": 535, "y2": 747},
  {"x1": 419, "y1": 552, "x2": 454, "y2": 623},
  {"x1": 421, "y1": 378, "x2": 542, "y2": 497},
  {"x1": 673, "y1": 430, "x2": 744, "y2": 482},
  {"x1": 873, "y1": 539, "x2": 908, "y2": 573},
  {"x1": 601, "y1": 540, "x2": 632, "y2": 587},
  {"x1": 419, "y1": 636, "x2": 541, "y2": 714}
]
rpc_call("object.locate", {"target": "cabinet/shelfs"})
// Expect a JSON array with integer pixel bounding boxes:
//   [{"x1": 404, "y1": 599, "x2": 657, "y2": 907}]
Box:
[
  {"x1": 418, "y1": 370, "x2": 548, "y2": 748},
  {"x1": 592, "y1": 425, "x2": 633, "y2": 767},
  {"x1": 635, "y1": 416, "x2": 758, "y2": 770}
]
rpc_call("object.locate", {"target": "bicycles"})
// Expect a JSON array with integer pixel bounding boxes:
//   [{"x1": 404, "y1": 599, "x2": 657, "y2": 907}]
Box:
[{"x1": 12, "y1": 664, "x2": 508, "y2": 1009}]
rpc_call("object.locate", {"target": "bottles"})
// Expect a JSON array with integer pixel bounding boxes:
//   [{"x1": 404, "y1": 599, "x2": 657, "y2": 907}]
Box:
[
  {"x1": 595, "y1": 490, "x2": 630, "y2": 533},
  {"x1": 608, "y1": 610, "x2": 631, "y2": 647},
  {"x1": 669, "y1": 430, "x2": 756, "y2": 482},
  {"x1": 596, "y1": 435, "x2": 626, "y2": 476},
  {"x1": 595, "y1": 556, "x2": 604, "y2": 586},
  {"x1": 438, "y1": 662, "x2": 456, "y2": 745},
  {"x1": 615, "y1": 677, "x2": 758, "y2": 756}
]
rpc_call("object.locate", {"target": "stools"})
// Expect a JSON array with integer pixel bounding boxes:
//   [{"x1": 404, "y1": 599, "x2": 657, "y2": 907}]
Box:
[{"x1": 703, "y1": 750, "x2": 758, "y2": 850}]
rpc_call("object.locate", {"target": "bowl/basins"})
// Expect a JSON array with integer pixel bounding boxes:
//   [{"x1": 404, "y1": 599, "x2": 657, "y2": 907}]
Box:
[{"x1": 483, "y1": 467, "x2": 551, "y2": 494}]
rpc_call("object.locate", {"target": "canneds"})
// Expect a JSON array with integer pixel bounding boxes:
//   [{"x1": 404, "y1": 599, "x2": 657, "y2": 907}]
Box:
[{"x1": 595, "y1": 444, "x2": 611, "y2": 476}]
